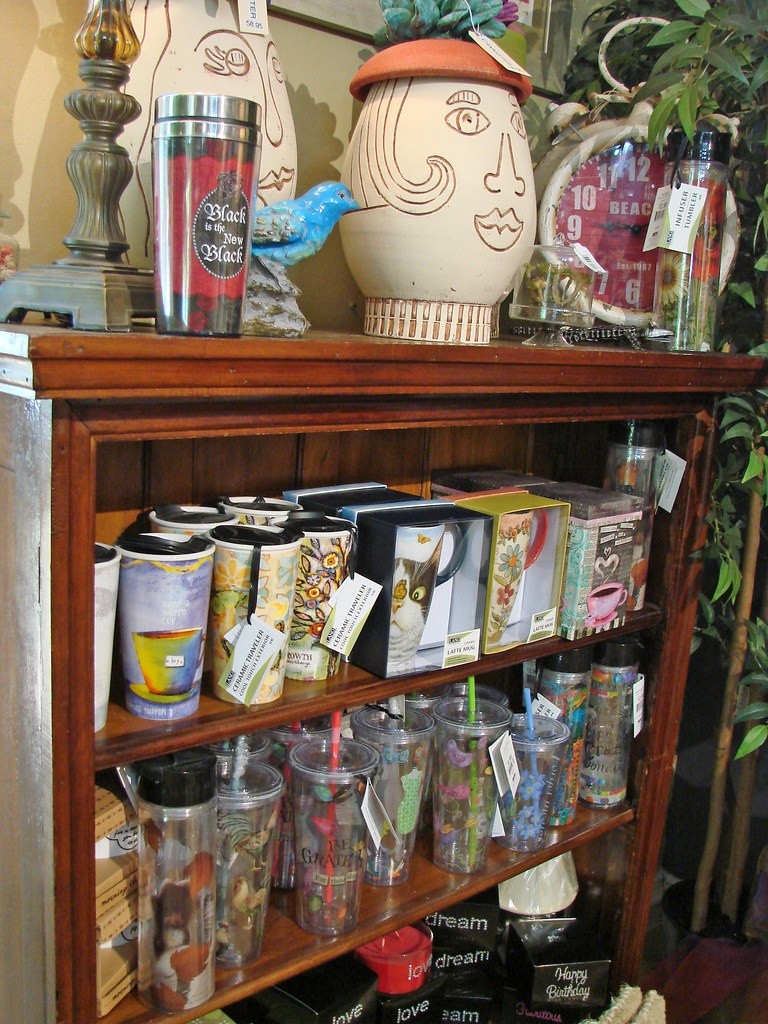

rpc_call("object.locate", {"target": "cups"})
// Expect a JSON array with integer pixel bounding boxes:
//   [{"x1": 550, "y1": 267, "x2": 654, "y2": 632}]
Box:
[
  {"x1": 450, "y1": 681, "x2": 508, "y2": 705},
  {"x1": 207, "y1": 734, "x2": 271, "y2": 760},
  {"x1": 151, "y1": 93, "x2": 263, "y2": 338},
  {"x1": 350, "y1": 703, "x2": 435, "y2": 886},
  {"x1": 95, "y1": 496, "x2": 357, "y2": 733},
  {"x1": 486, "y1": 509, "x2": 548, "y2": 645},
  {"x1": 536, "y1": 647, "x2": 592, "y2": 825},
  {"x1": 396, "y1": 684, "x2": 452, "y2": 837},
  {"x1": 137, "y1": 747, "x2": 217, "y2": 1013},
  {"x1": 433, "y1": 696, "x2": 514, "y2": 873},
  {"x1": 267, "y1": 714, "x2": 334, "y2": 892},
  {"x1": 386, "y1": 523, "x2": 468, "y2": 666},
  {"x1": 605, "y1": 417, "x2": 660, "y2": 611},
  {"x1": 491, "y1": 713, "x2": 571, "y2": 852},
  {"x1": 578, "y1": 640, "x2": 640, "y2": 810},
  {"x1": 651, "y1": 130, "x2": 733, "y2": 354},
  {"x1": 289, "y1": 736, "x2": 383, "y2": 935},
  {"x1": 215, "y1": 760, "x2": 287, "y2": 969}
]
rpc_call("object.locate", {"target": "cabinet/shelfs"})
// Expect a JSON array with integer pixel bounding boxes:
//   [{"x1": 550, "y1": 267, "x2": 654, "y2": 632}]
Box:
[{"x1": 0, "y1": 322, "x2": 768, "y2": 1024}]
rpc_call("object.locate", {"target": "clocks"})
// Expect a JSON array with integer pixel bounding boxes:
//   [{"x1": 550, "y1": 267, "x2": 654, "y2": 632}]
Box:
[{"x1": 533, "y1": 16, "x2": 742, "y2": 346}]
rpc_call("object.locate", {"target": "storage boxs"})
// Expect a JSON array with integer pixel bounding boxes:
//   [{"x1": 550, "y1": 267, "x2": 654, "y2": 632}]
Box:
[
  {"x1": 180, "y1": 885, "x2": 613, "y2": 1024},
  {"x1": 278, "y1": 468, "x2": 646, "y2": 678},
  {"x1": 94, "y1": 781, "x2": 158, "y2": 1019}
]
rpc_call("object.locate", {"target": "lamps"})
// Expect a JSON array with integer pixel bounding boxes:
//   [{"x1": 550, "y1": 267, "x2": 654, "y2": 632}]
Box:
[{"x1": 0, "y1": 0, "x2": 156, "y2": 333}]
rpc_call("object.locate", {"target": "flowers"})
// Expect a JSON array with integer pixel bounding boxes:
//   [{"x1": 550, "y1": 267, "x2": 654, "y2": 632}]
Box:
[{"x1": 657, "y1": 251, "x2": 691, "y2": 304}]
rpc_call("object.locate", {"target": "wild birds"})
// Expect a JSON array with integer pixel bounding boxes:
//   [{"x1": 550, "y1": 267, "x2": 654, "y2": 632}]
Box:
[{"x1": 248, "y1": 180, "x2": 363, "y2": 267}]
